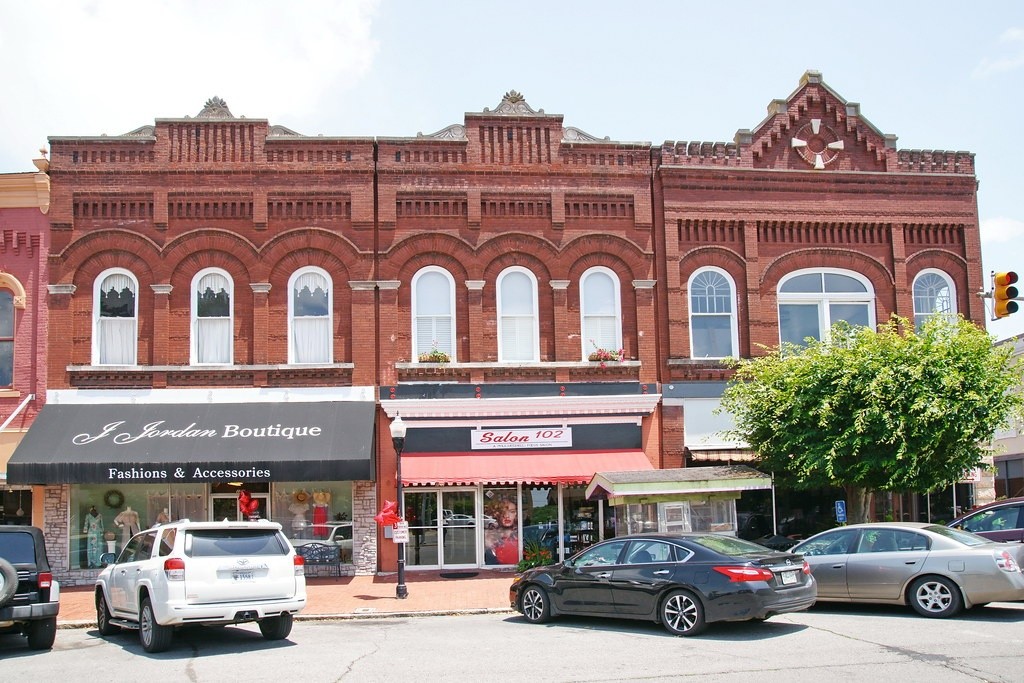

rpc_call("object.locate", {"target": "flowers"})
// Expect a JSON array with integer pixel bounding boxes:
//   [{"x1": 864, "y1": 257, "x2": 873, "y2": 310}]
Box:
[
  {"x1": 419, "y1": 341, "x2": 446, "y2": 366},
  {"x1": 586, "y1": 338, "x2": 625, "y2": 368},
  {"x1": 516, "y1": 540, "x2": 554, "y2": 574}
]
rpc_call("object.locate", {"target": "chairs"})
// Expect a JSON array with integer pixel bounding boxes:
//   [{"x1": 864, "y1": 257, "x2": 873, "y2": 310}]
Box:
[
  {"x1": 631, "y1": 551, "x2": 652, "y2": 562},
  {"x1": 667, "y1": 551, "x2": 686, "y2": 561},
  {"x1": 884, "y1": 537, "x2": 900, "y2": 551}
]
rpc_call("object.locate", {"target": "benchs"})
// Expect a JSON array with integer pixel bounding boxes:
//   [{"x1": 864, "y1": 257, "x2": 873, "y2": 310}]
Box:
[{"x1": 293, "y1": 542, "x2": 342, "y2": 581}]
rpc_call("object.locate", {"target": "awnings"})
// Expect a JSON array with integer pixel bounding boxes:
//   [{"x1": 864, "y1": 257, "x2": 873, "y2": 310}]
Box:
[
  {"x1": 399, "y1": 452, "x2": 654, "y2": 485},
  {"x1": 6, "y1": 385, "x2": 376, "y2": 484}
]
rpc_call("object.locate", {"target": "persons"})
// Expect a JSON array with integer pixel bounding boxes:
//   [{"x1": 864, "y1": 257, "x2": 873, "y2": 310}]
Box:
[
  {"x1": 312, "y1": 491, "x2": 330, "y2": 537},
  {"x1": 156, "y1": 507, "x2": 176, "y2": 544},
  {"x1": 433, "y1": 509, "x2": 447, "y2": 546},
  {"x1": 83, "y1": 507, "x2": 105, "y2": 569},
  {"x1": 114, "y1": 506, "x2": 141, "y2": 550},
  {"x1": 484, "y1": 497, "x2": 528, "y2": 565},
  {"x1": 288, "y1": 490, "x2": 310, "y2": 530}
]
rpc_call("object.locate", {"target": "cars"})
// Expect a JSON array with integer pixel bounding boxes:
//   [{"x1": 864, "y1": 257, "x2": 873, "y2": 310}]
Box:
[
  {"x1": 286, "y1": 522, "x2": 353, "y2": 559},
  {"x1": 784, "y1": 522, "x2": 1024, "y2": 619},
  {"x1": 430, "y1": 509, "x2": 499, "y2": 529},
  {"x1": 944, "y1": 497, "x2": 1024, "y2": 545},
  {"x1": 507, "y1": 531, "x2": 819, "y2": 637}
]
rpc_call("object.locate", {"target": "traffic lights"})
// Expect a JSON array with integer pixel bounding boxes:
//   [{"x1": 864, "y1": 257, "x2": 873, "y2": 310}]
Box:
[{"x1": 994, "y1": 269, "x2": 1019, "y2": 319}]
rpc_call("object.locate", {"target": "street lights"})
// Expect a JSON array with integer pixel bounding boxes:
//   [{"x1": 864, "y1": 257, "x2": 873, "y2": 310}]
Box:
[{"x1": 388, "y1": 410, "x2": 411, "y2": 600}]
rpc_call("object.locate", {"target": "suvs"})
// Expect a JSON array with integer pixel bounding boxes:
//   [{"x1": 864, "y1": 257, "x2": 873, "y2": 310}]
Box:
[
  {"x1": 93, "y1": 518, "x2": 309, "y2": 653},
  {"x1": 0, "y1": 525, "x2": 60, "y2": 650}
]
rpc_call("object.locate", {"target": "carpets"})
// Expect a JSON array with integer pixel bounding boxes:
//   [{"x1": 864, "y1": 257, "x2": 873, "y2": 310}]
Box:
[{"x1": 440, "y1": 572, "x2": 480, "y2": 578}]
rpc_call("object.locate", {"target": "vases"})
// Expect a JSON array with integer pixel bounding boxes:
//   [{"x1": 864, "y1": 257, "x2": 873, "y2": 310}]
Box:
[
  {"x1": 589, "y1": 355, "x2": 616, "y2": 361},
  {"x1": 420, "y1": 355, "x2": 450, "y2": 363}
]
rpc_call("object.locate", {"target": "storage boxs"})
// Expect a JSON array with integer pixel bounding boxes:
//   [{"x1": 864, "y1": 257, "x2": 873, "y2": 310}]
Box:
[{"x1": 709, "y1": 524, "x2": 733, "y2": 532}]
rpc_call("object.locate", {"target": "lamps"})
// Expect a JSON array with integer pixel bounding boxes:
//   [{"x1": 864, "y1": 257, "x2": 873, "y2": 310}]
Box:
[{"x1": 9, "y1": 486, "x2": 13, "y2": 492}]
